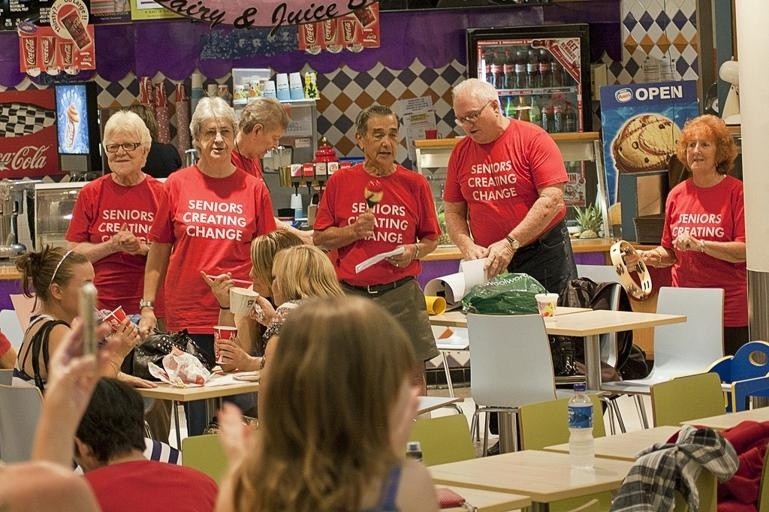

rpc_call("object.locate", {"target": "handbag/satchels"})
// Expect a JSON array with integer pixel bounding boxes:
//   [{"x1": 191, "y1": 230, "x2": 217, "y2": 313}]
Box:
[{"x1": 121, "y1": 328, "x2": 216, "y2": 380}]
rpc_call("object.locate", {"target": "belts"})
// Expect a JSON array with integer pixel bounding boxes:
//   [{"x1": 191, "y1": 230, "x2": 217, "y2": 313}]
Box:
[{"x1": 341, "y1": 274, "x2": 415, "y2": 294}]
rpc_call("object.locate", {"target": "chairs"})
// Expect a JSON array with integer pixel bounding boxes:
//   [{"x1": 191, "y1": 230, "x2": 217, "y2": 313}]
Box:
[
  {"x1": 0, "y1": 383, "x2": 44, "y2": 469},
  {"x1": 650, "y1": 373, "x2": 727, "y2": 434},
  {"x1": 756, "y1": 445, "x2": 769, "y2": 510},
  {"x1": 407, "y1": 395, "x2": 466, "y2": 422},
  {"x1": 692, "y1": 473, "x2": 718, "y2": 510},
  {"x1": 596, "y1": 282, "x2": 628, "y2": 434},
  {"x1": 518, "y1": 395, "x2": 615, "y2": 512},
  {"x1": 705, "y1": 340, "x2": 769, "y2": 413},
  {"x1": 0, "y1": 369, "x2": 14, "y2": 387},
  {"x1": 180, "y1": 430, "x2": 235, "y2": 485},
  {"x1": 398, "y1": 413, "x2": 477, "y2": 468},
  {"x1": 629, "y1": 285, "x2": 726, "y2": 433},
  {"x1": 466, "y1": 312, "x2": 560, "y2": 458},
  {"x1": 428, "y1": 326, "x2": 468, "y2": 398}
]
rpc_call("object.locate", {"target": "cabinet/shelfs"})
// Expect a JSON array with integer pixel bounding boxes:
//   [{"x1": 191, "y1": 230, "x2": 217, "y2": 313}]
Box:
[{"x1": 413, "y1": 130, "x2": 610, "y2": 249}]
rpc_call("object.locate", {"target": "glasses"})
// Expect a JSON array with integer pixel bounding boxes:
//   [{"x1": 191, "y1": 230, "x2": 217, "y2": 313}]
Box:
[
  {"x1": 105, "y1": 142, "x2": 142, "y2": 153},
  {"x1": 455, "y1": 99, "x2": 492, "y2": 127}
]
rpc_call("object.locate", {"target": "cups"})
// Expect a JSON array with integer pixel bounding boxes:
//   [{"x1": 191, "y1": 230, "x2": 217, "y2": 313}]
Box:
[
  {"x1": 102, "y1": 305, "x2": 132, "y2": 336},
  {"x1": 229, "y1": 286, "x2": 259, "y2": 318},
  {"x1": 213, "y1": 324, "x2": 239, "y2": 366},
  {"x1": 534, "y1": 292, "x2": 560, "y2": 320}
]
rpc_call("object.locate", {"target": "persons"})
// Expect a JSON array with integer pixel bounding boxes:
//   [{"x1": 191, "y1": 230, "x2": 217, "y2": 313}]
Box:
[
  {"x1": 138, "y1": 95, "x2": 276, "y2": 437},
  {"x1": 200, "y1": 229, "x2": 306, "y2": 423},
  {"x1": 311, "y1": 104, "x2": 441, "y2": 398},
  {"x1": 1, "y1": 327, "x2": 17, "y2": 371},
  {"x1": 16, "y1": 243, "x2": 187, "y2": 467},
  {"x1": 625, "y1": 113, "x2": 750, "y2": 415},
  {"x1": 1, "y1": 310, "x2": 121, "y2": 512},
  {"x1": 72, "y1": 376, "x2": 222, "y2": 512},
  {"x1": 231, "y1": 97, "x2": 292, "y2": 193},
  {"x1": 228, "y1": 245, "x2": 348, "y2": 427},
  {"x1": 214, "y1": 294, "x2": 441, "y2": 511},
  {"x1": 443, "y1": 77, "x2": 578, "y2": 389},
  {"x1": 63, "y1": 109, "x2": 174, "y2": 332},
  {"x1": 123, "y1": 104, "x2": 183, "y2": 177}
]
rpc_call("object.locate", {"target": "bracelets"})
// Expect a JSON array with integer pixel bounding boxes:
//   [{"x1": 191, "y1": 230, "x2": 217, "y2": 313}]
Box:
[
  {"x1": 109, "y1": 359, "x2": 121, "y2": 378},
  {"x1": 652, "y1": 248, "x2": 663, "y2": 271},
  {"x1": 699, "y1": 240, "x2": 706, "y2": 253},
  {"x1": 219, "y1": 306, "x2": 232, "y2": 311}
]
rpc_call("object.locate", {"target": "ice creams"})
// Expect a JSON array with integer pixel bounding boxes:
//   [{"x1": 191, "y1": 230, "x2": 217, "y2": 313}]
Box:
[{"x1": 64, "y1": 103, "x2": 79, "y2": 146}]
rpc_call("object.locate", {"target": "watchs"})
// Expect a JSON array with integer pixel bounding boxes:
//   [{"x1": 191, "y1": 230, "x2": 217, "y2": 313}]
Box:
[
  {"x1": 139, "y1": 299, "x2": 155, "y2": 310},
  {"x1": 506, "y1": 235, "x2": 524, "y2": 252},
  {"x1": 414, "y1": 243, "x2": 420, "y2": 260}
]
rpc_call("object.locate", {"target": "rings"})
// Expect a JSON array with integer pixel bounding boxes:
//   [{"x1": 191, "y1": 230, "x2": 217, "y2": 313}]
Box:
[{"x1": 394, "y1": 263, "x2": 399, "y2": 267}]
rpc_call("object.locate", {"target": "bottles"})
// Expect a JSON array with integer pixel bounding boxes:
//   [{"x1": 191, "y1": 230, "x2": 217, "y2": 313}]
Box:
[
  {"x1": 563, "y1": 102, "x2": 577, "y2": 132},
  {"x1": 563, "y1": 66, "x2": 571, "y2": 86},
  {"x1": 541, "y1": 106, "x2": 553, "y2": 132},
  {"x1": 515, "y1": 51, "x2": 526, "y2": 88},
  {"x1": 485, "y1": 51, "x2": 490, "y2": 83},
  {"x1": 539, "y1": 49, "x2": 550, "y2": 88},
  {"x1": 567, "y1": 382, "x2": 595, "y2": 471},
  {"x1": 552, "y1": 106, "x2": 563, "y2": 132},
  {"x1": 503, "y1": 50, "x2": 515, "y2": 89},
  {"x1": 551, "y1": 59, "x2": 561, "y2": 87},
  {"x1": 499, "y1": 97, "x2": 505, "y2": 115},
  {"x1": 527, "y1": 50, "x2": 539, "y2": 88},
  {"x1": 490, "y1": 50, "x2": 503, "y2": 89},
  {"x1": 528, "y1": 96, "x2": 541, "y2": 126},
  {"x1": 505, "y1": 96, "x2": 516, "y2": 118},
  {"x1": 517, "y1": 96, "x2": 529, "y2": 121}
]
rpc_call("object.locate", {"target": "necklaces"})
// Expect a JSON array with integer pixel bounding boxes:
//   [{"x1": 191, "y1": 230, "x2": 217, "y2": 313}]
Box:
[{"x1": 689, "y1": 174, "x2": 725, "y2": 186}]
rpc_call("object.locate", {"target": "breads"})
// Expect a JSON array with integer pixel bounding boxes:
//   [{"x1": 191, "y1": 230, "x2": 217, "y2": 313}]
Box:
[{"x1": 611, "y1": 112, "x2": 679, "y2": 173}]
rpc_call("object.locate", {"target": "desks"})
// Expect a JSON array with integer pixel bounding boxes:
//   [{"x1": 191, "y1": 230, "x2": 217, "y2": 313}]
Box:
[
  {"x1": 541, "y1": 424, "x2": 682, "y2": 463},
  {"x1": 426, "y1": 447, "x2": 638, "y2": 510},
  {"x1": 429, "y1": 307, "x2": 595, "y2": 330},
  {"x1": 542, "y1": 309, "x2": 687, "y2": 390},
  {"x1": 678, "y1": 406, "x2": 769, "y2": 435},
  {"x1": 431, "y1": 483, "x2": 534, "y2": 512},
  {"x1": 133, "y1": 370, "x2": 259, "y2": 450}
]
